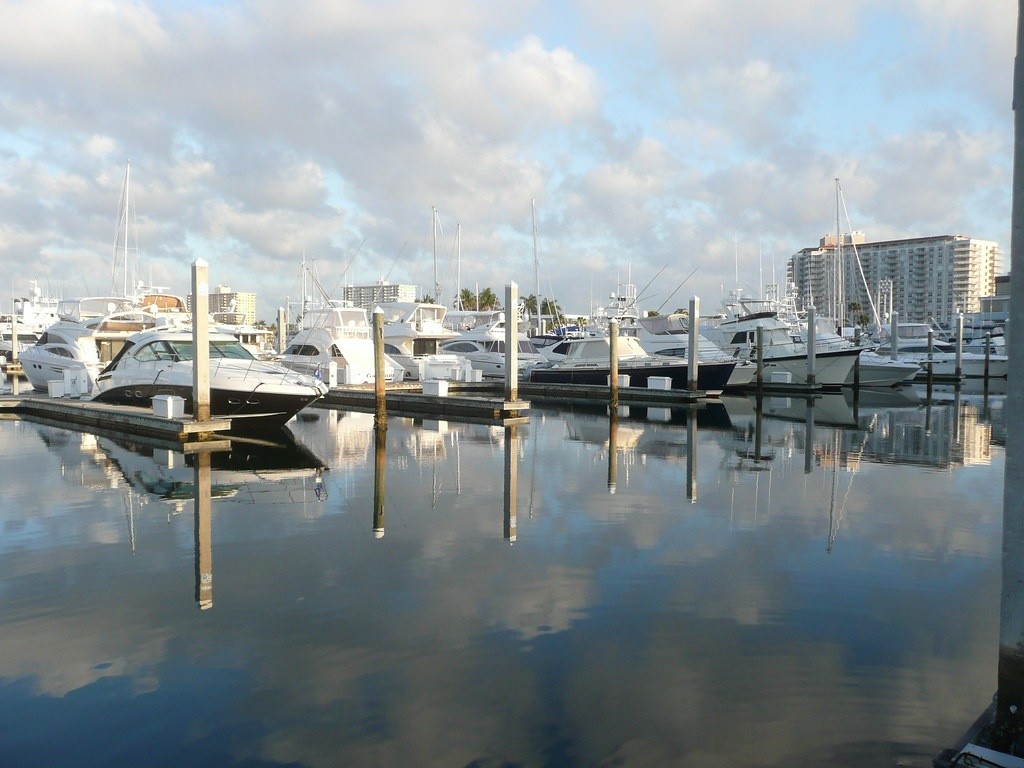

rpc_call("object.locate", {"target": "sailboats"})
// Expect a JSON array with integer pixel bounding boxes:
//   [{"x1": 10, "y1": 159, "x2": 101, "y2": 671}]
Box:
[{"x1": 21, "y1": 157, "x2": 1006, "y2": 397}]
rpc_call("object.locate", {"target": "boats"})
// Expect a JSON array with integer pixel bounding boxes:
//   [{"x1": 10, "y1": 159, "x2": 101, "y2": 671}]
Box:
[
  {"x1": 96, "y1": 435, "x2": 331, "y2": 505},
  {"x1": 90, "y1": 329, "x2": 330, "y2": 430}
]
